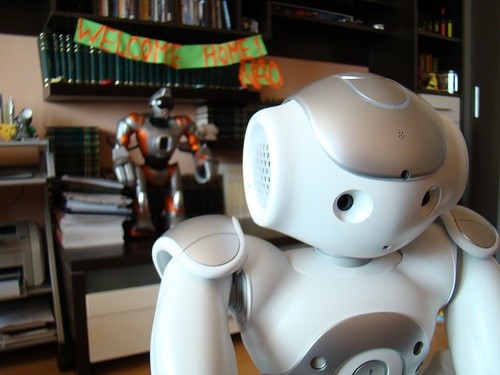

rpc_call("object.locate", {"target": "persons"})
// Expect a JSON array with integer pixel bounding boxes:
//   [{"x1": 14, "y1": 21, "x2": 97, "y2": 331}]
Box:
[
  {"x1": 112, "y1": 88, "x2": 221, "y2": 266},
  {"x1": 149, "y1": 72, "x2": 499, "y2": 375}
]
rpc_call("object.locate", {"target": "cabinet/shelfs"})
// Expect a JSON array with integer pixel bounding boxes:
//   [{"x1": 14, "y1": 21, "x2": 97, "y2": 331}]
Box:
[{"x1": 0, "y1": 0, "x2": 472, "y2": 372}]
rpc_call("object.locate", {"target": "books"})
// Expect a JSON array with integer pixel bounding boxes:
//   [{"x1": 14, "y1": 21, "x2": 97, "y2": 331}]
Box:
[
  {"x1": 1, "y1": 299, "x2": 56, "y2": 348},
  {"x1": 34, "y1": 20, "x2": 243, "y2": 96},
  {"x1": 0, "y1": 163, "x2": 33, "y2": 180},
  {"x1": 417, "y1": 2, "x2": 456, "y2": 39},
  {"x1": 418, "y1": 53, "x2": 447, "y2": 92},
  {"x1": 99, "y1": 0, "x2": 225, "y2": 29}
]
